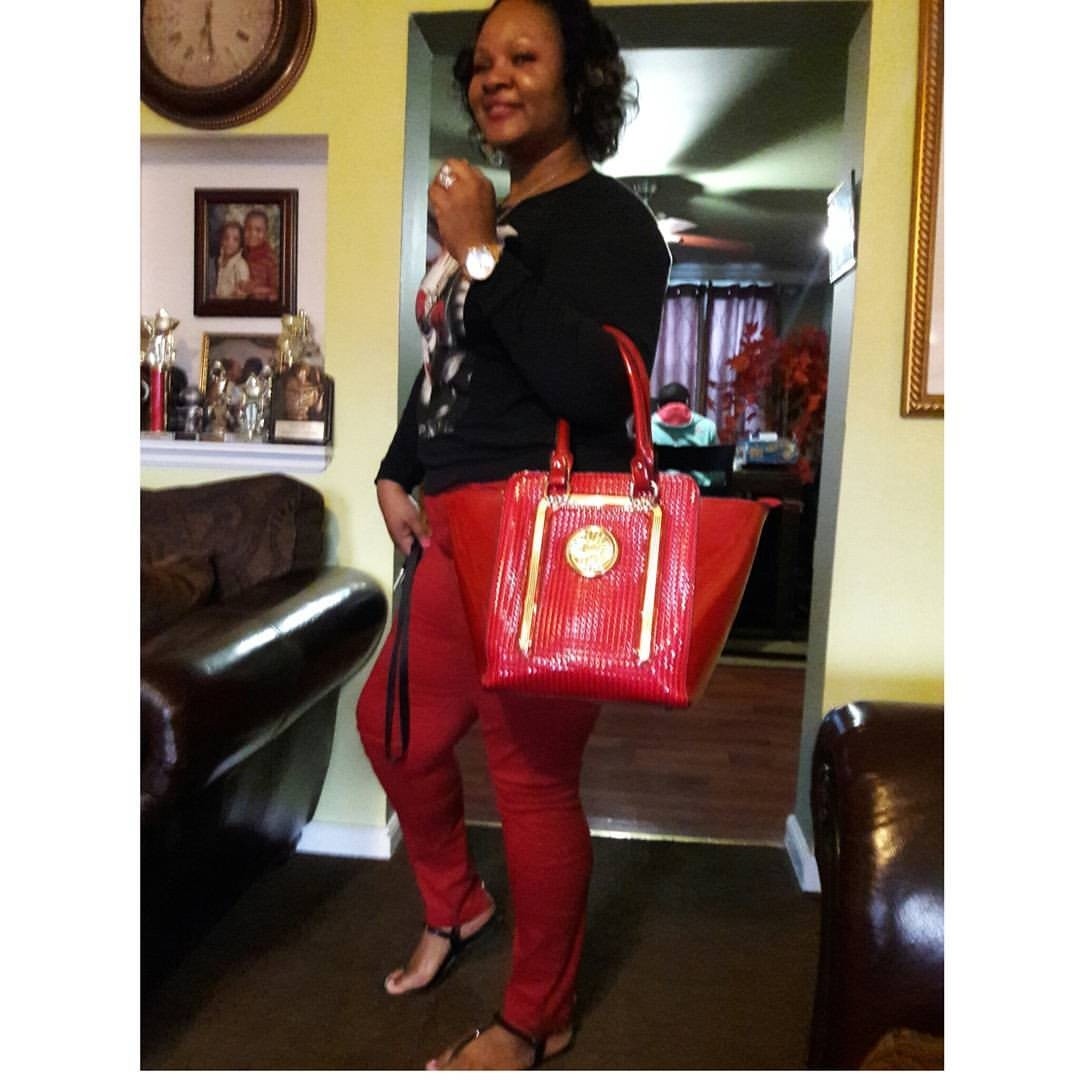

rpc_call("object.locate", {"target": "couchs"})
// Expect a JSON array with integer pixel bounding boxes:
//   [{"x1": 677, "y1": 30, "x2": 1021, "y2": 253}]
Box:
[
  {"x1": 789, "y1": 691, "x2": 944, "y2": 1070},
  {"x1": 140, "y1": 467, "x2": 392, "y2": 1020}
]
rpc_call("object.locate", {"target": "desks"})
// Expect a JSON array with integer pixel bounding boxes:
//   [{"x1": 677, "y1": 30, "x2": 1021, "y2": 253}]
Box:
[{"x1": 731, "y1": 468, "x2": 804, "y2": 644}]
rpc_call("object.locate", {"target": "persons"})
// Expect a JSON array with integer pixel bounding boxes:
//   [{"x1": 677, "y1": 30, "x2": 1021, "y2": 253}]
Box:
[
  {"x1": 649, "y1": 382, "x2": 722, "y2": 448},
  {"x1": 357, "y1": 0, "x2": 768, "y2": 1070},
  {"x1": 214, "y1": 210, "x2": 278, "y2": 302},
  {"x1": 217, "y1": 356, "x2": 264, "y2": 392}
]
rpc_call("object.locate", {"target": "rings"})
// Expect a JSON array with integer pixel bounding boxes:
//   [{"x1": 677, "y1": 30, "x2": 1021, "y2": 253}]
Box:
[{"x1": 435, "y1": 165, "x2": 457, "y2": 189}]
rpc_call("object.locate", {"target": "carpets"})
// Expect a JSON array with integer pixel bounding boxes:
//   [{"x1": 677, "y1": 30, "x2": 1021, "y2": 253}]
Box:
[{"x1": 141, "y1": 827, "x2": 823, "y2": 1070}]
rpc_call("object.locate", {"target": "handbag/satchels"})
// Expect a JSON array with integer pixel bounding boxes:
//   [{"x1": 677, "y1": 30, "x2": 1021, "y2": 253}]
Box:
[{"x1": 483, "y1": 325, "x2": 782, "y2": 710}]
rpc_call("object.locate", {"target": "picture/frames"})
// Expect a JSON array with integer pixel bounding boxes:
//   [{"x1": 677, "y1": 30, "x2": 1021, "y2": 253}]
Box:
[
  {"x1": 898, "y1": 0, "x2": 944, "y2": 418},
  {"x1": 199, "y1": 328, "x2": 280, "y2": 396},
  {"x1": 192, "y1": 185, "x2": 301, "y2": 321}
]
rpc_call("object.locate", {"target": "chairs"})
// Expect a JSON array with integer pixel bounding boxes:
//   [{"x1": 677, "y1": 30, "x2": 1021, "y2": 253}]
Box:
[{"x1": 653, "y1": 444, "x2": 740, "y2": 499}]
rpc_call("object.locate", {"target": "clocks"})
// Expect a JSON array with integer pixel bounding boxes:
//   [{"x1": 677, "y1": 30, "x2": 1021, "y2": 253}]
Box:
[{"x1": 140, "y1": 0, "x2": 322, "y2": 132}]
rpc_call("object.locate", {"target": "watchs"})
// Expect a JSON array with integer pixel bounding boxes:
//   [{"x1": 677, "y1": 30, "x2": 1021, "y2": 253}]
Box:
[{"x1": 459, "y1": 240, "x2": 502, "y2": 282}]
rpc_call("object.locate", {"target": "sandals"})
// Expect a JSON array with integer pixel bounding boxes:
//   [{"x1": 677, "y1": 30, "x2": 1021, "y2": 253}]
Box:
[
  {"x1": 424, "y1": 1008, "x2": 577, "y2": 1071},
  {"x1": 382, "y1": 875, "x2": 508, "y2": 997}
]
